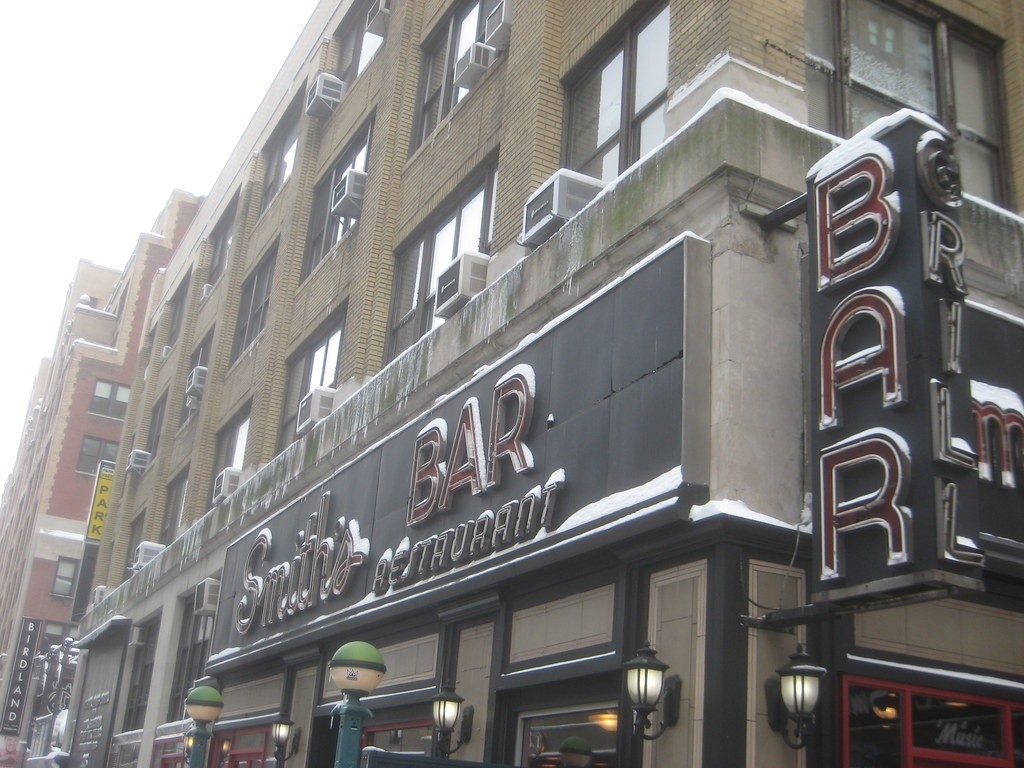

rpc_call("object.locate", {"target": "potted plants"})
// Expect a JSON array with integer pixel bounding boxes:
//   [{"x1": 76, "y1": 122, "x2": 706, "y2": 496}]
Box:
[{"x1": 559, "y1": 736, "x2": 592, "y2": 768}]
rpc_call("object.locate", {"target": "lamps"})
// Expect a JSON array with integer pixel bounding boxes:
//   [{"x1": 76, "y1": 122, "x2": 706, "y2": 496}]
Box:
[
  {"x1": 431, "y1": 680, "x2": 473, "y2": 755},
  {"x1": 184, "y1": 724, "x2": 231, "y2": 768},
  {"x1": 270, "y1": 712, "x2": 301, "y2": 761},
  {"x1": 622, "y1": 640, "x2": 683, "y2": 740},
  {"x1": 765, "y1": 644, "x2": 826, "y2": 748}
]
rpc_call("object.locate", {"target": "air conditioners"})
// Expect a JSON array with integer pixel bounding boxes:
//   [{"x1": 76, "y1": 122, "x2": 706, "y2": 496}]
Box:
[
  {"x1": 304, "y1": 72, "x2": 342, "y2": 119},
  {"x1": 91, "y1": 585, "x2": 106, "y2": 607},
  {"x1": 133, "y1": 541, "x2": 167, "y2": 570},
  {"x1": 520, "y1": 168, "x2": 604, "y2": 250},
  {"x1": 434, "y1": 251, "x2": 488, "y2": 318},
  {"x1": 452, "y1": 0, "x2": 512, "y2": 90},
  {"x1": 161, "y1": 345, "x2": 171, "y2": 359},
  {"x1": 213, "y1": 467, "x2": 239, "y2": 503},
  {"x1": 144, "y1": 366, "x2": 149, "y2": 380},
  {"x1": 153, "y1": 267, "x2": 164, "y2": 278},
  {"x1": 193, "y1": 577, "x2": 219, "y2": 615},
  {"x1": 185, "y1": 366, "x2": 208, "y2": 396},
  {"x1": 200, "y1": 284, "x2": 213, "y2": 301},
  {"x1": 296, "y1": 385, "x2": 333, "y2": 436},
  {"x1": 331, "y1": 169, "x2": 366, "y2": 216},
  {"x1": 126, "y1": 449, "x2": 151, "y2": 473},
  {"x1": 366, "y1": 0, "x2": 390, "y2": 34}
]
